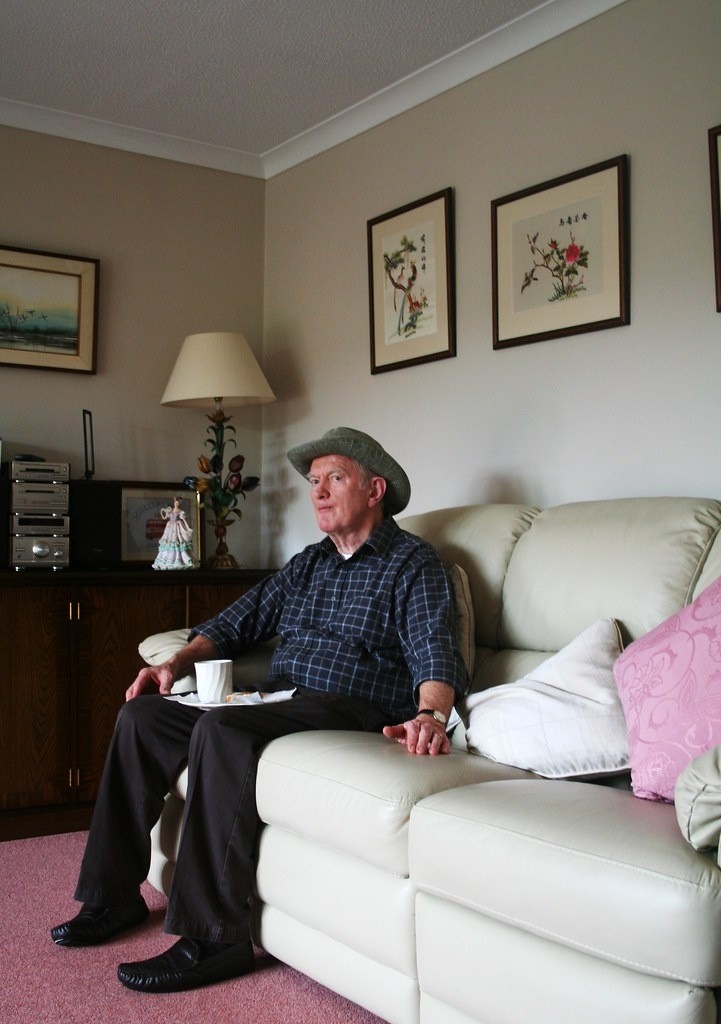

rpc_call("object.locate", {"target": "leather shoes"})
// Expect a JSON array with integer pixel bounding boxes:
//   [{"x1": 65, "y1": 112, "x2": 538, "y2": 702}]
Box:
[
  {"x1": 117, "y1": 938, "x2": 254, "y2": 992},
  {"x1": 49, "y1": 891, "x2": 150, "y2": 945}
]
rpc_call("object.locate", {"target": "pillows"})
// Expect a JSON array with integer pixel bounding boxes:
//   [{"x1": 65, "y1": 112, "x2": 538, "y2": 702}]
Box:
[
  {"x1": 614, "y1": 574, "x2": 721, "y2": 799},
  {"x1": 447, "y1": 562, "x2": 475, "y2": 680},
  {"x1": 445, "y1": 617, "x2": 632, "y2": 781}
]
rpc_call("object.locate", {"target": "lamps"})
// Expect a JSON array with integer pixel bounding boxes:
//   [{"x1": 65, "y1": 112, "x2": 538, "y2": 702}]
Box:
[{"x1": 160, "y1": 332, "x2": 275, "y2": 568}]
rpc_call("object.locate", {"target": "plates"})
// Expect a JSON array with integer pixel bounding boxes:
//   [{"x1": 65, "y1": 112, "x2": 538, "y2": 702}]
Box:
[{"x1": 179, "y1": 693, "x2": 293, "y2": 711}]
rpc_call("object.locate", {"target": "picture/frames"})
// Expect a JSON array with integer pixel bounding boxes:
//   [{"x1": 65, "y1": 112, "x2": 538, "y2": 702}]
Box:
[
  {"x1": 116, "y1": 480, "x2": 206, "y2": 567},
  {"x1": 0, "y1": 246, "x2": 100, "y2": 376},
  {"x1": 366, "y1": 188, "x2": 457, "y2": 375},
  {"x1": 490, "y1": 154, "x2": 630, "y2": 349}
]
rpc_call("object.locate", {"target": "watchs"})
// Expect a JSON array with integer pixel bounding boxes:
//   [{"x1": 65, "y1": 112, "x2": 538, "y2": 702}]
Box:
[{"x1": 418, "y1": 708, "x2": 447, "y2": 726}]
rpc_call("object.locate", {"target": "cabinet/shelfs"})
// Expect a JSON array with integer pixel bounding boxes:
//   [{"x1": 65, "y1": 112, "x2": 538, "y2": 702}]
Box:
[{"x1": 0, "y1": 569, "x2": 283, "y2": 839}]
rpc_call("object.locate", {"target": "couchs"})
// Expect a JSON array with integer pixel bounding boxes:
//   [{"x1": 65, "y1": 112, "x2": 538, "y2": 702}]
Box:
[{"x1": 137, "y1": 495, "x2": 721, "y2": 1024}]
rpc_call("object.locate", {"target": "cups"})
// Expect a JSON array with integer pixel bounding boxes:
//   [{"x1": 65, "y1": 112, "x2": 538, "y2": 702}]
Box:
[{"x1": 194, "y1": 660, "x2": 233, "y2": 702}]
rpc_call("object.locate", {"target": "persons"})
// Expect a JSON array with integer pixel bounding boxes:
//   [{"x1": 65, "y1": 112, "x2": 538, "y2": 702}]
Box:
[
  {"x1": 52, "y1": 426, "x2": 470, "y2": 993},
  {"x1": 151, "y1": 496, "x2": 200, "y2": 570}
]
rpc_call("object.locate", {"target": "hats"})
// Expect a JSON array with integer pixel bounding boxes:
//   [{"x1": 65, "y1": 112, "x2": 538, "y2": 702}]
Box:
[{"x1": 288, "y1": 427, "x2": 411, "y2": 516}]
